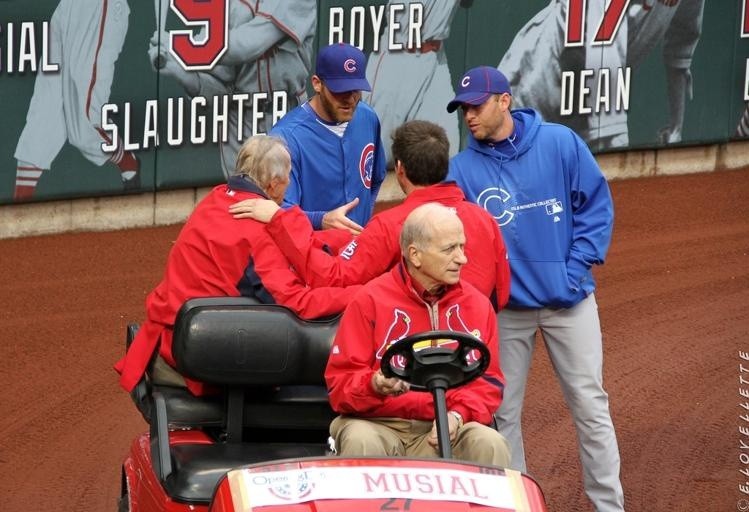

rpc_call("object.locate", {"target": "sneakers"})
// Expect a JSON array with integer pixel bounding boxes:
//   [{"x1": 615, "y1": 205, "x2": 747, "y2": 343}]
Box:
[{"x1": 122, "y1": 152, "x2": 143, "y2": 193}]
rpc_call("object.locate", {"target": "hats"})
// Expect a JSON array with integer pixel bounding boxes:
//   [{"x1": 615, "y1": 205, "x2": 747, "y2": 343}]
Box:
[
  {"x1": 315, "y1": 42, "x2": 372, "y2": 95},
  {"x1": 445, "y1": 65, "x2": 512, "y2": 115}
]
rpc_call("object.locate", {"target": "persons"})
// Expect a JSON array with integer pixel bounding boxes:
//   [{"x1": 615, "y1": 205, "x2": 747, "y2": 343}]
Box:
[
  {"x1": 446, "y1": 64, "x2": 628, "y2": 512},
  {"x1": 360, "y1": 0, "x2": 463, "y2": 167},
  {"x1": 322, "y1": 201, "x2": 513, "y2": 468},
  {"x1": 495, "y1": 0, "x2": 706, "y2": 154},
  {"x1": 147, "y1": 0, "x2": 319, "y2": 185},
  {"x1": 113, "y1": 133, "x2": 370, "y2": 425},
  {"x1": 263, "y1": 42, "x2": 389, "y2": 233},
  {"x1": 10, "y1": 1, "x2": 141, "y2": 198},
  {"x1": 228, "y1": 120, "x2": 513, "y2": 312}
]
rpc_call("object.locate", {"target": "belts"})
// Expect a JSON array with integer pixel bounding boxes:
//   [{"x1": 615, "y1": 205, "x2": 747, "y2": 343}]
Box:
[{"x1": 399, "y1": 38, "x2": 443, "y2": 54}]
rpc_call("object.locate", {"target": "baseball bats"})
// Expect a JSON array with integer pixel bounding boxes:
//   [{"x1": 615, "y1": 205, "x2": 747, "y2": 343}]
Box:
[{"x1": 154, "y1": 0, "x2": 170, "y2": 69}]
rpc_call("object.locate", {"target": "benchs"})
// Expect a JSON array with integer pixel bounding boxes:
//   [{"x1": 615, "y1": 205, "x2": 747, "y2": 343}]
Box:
[{"x1": 117, "y1": 296, "x2": 342, "y2": 500}]
